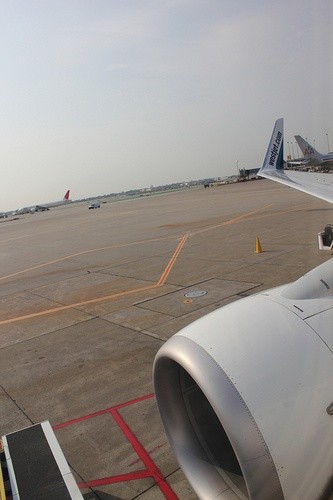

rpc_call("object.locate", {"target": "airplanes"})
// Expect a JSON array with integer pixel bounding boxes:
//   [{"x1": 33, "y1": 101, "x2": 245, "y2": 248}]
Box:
[
  {"x1": 1, "y1": 117, "x2": 332, "y2": 500},
  {"x1": 88, "y1": 200, "x2": 100, "y2": 209},
  {"x1": 0, "y1": 205, "x2": 50, "y2": 218},
  {"x1": 287, "y1": 135, "x2": 333, "y2": 167}
]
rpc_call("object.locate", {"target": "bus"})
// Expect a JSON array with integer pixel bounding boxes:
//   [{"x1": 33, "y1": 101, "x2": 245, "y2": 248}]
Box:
[{"x1": 239, "y1": 168, "x2": 261, "y2": 180}]
